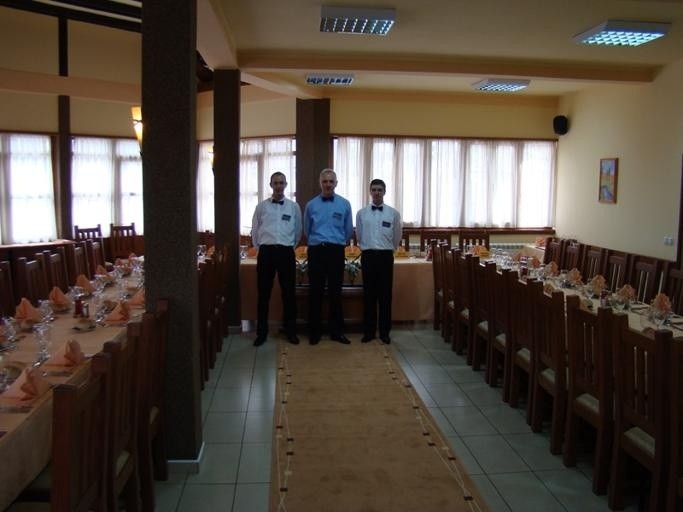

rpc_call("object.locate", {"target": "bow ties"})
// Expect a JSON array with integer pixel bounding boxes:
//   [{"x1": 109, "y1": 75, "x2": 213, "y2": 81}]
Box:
[
  {"x1": 271, "y1": 199, "x2": 284, "y2": 205},
  {"x1": 321, "y1": 196, "x2": 334, "y2": 202},
  {"x1": 372, "y1": 205, "x2": 383, "y2": 212}
]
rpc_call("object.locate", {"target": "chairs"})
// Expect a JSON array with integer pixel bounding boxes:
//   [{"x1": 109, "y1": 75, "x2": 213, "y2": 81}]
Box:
[
  {"x1": 346, "y1": 229, "x2": 357, "y2": 247},
  {"x1": 295, "y1": 235, "x2": 308, "y2": 250}
]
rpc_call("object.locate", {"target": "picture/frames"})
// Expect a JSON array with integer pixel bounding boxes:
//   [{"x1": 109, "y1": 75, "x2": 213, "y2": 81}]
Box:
[{"x1": 598, "y1": 158, "x2": 618, "y2": 203}]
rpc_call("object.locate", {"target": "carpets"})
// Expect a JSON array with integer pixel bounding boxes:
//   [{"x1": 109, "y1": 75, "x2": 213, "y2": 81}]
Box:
[{"x1": 268, "y1": 331, "x2": 492, "y2": 512}]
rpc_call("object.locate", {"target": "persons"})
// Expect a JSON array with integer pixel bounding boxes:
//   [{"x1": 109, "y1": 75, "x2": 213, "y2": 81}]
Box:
[
  {"x1": 251, "y1": 171, "x2": 303, "y2": 346},
  {"x1": 355, "y1": 179, "x2": 403, "y2": 344},
  {"x1": 303, "y1": 168, "x2": 352, "y2": 345}
]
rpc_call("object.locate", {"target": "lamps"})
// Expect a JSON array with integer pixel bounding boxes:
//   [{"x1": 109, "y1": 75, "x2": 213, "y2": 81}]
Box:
[
  {"x1": 570, "y1": 20, "x2": 672, "y2": 47},
  {"x1": 317, "y1": 5, "x2": 398, "y2": 37},
  {"x1": 305, "y1": 73, "x2": 357, "y2": 86},
  {"x1": 472, "y1": 78, "x2": 530, "y2": 93}
]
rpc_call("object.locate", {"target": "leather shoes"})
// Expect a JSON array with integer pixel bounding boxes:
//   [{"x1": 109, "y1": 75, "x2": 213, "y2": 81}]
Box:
[
  {"x1": 253, "y1": 334, "x2": 267, "y2": 346},
  {"x1": 382, "y1": 336, "x2": 391, "y2": 344},
  {"x1": 361, "y1": 333, "x2": 376, "y2": 343},
  {"x1": 280, "y1": 331, "x2": 299, "y2": 344},
  {"x1": 309, "y1": 333, "x2": 320, "y2": 345},
  {"x1": 329, "y1": 333, "x2": 351, "y2": 344}
]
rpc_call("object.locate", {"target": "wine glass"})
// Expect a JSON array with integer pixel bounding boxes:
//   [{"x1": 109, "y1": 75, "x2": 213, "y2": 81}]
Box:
[
  {"x1": 197, "y1": 244, "x2": 206, "y2": 262},
  {"x1": 239, "y1": 244, "x2": 248, "y2": 262},
  {"x1": 1, "y1": 259, "x2": 141, "y2": 366},
  {"x1": 458, "y1": 245, "x2": 673, "y2": 330}
]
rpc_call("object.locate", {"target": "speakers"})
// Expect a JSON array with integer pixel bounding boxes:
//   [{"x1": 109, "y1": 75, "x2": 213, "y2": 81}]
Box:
[{"x1": 553, "y1": 115, "x2": 567, "y2": 134}]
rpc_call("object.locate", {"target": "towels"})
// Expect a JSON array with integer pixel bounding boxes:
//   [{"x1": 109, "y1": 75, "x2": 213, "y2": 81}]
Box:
[
  {"x1": 295, "y1": 246, "x2": 308, "y2": 258},
  {"x1": 344, "y1": 246, "x2": 361, "y2": 257},
  {"x1": 248, "y1": 246, "x2": 256, "y2": 256},
  {"x1": 395, "y1": 245, "x2": 413, "y2": 257}
]
